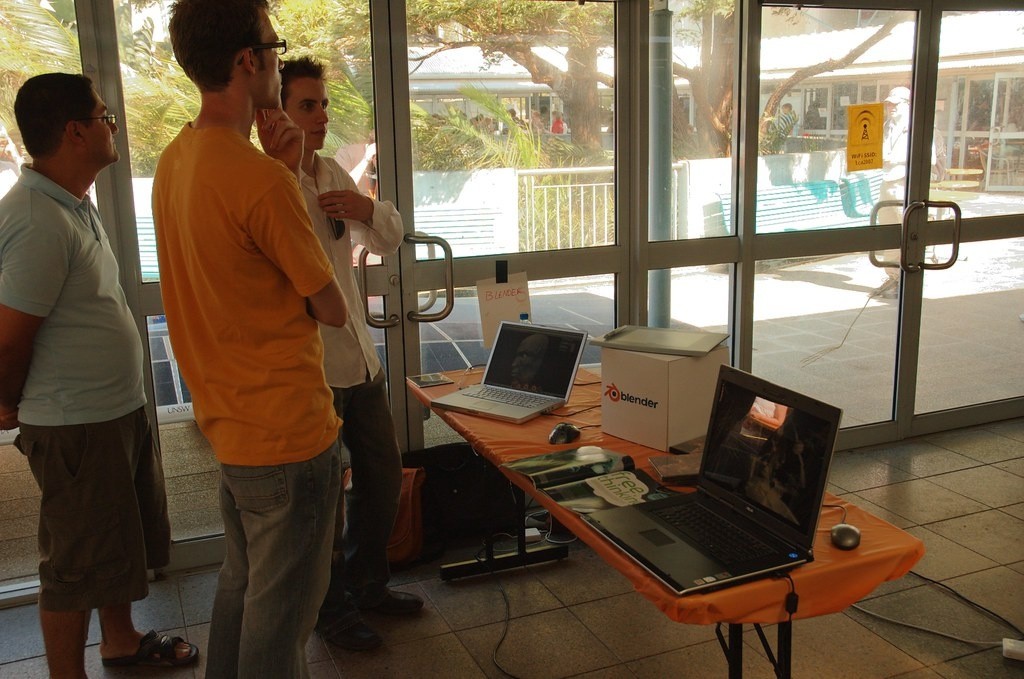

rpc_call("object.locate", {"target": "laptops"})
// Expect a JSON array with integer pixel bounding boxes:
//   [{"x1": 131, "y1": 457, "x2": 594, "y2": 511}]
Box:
[
  {"x1": 580, "y1": 365, "x2": 845, "y2": 598},
  {"x1": 430, "y1": 321, "x2": 589, "y2": 424}
]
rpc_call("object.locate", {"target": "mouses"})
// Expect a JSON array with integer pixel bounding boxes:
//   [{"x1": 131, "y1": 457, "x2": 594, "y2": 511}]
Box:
[
  {"x1": 548, "y1": 422, "x2": 581, "y2": 445},
  {"x1": 830, "y1": 522, "x2": 861, "y2": 550}
]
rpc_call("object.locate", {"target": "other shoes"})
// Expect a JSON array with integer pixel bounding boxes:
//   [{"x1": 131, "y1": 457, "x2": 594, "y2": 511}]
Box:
[
  {"x1": 872, "y1": 278, "x2": 898, "y2": 295},
  {"x1": 882, "y1": 284, "x2": 899, "y2": 299}
]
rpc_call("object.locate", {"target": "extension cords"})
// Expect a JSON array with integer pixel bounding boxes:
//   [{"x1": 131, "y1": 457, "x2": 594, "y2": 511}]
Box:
[{"x1": 1002, "y1": 638, "x2": 1024, "y2": 661}]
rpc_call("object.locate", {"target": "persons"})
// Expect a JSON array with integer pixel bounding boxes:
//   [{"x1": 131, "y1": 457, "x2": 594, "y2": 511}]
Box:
[
  {"x1": 431, "y1": 113, "x2": 491, "y2": 133},
  {"x1": 761, "y1": 412, "x2": 827, "y2": 487},
  {"x1": 282, "y1": 56, "x2": 424, "y2": 652},
  {"x1": 152, "y1": 0, "x2": 348, "y2": 679},
  {"x1": 510, "y1": 335, "x2": 548, "y2": 392},
  {"x1": 552, "y1": 111, "x2": 564, "y2": 134},
  {"x1": 532, "y1": 106, "x2": 550, "y2": 131},
  {"x1": 0, "y1": 72, "x2": 197, "y2": 679},
  {"x1": 336, "y1": 130, "x2": 380, "y2": 265},
  {"x1": 779, "y1": 104, "x2": 793, "y2": 136},
  {"x1": 504, "y1": 109, "x2": 525, "y2": 133},
  {"x1": 870, "y1": 87, "x2": 934, "y2": 297},
  {"x1": 1, "y1": 134, "x2": 24, "y2": 162},
  {"x1": 807, "y1": 100, "x2": 822, "y2": 129}
]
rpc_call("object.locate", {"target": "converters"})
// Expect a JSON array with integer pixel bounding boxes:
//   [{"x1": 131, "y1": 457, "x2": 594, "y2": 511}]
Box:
[{"x1": 524, "y1": 527, "x2": 541, "y2": 542}]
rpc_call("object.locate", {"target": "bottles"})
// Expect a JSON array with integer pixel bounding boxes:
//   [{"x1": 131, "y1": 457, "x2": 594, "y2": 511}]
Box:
[{"x1": 520, "y1": 314, "x2": 531, "y2": 325}]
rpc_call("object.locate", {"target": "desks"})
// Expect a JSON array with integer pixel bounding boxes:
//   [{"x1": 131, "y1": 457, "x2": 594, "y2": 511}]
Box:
[
  {"x1": 407, "y1": 366, "x2": 923, "y2": 679},
  {"x1": 925, "y1": 169, "x2": 983, "y2": 261}
]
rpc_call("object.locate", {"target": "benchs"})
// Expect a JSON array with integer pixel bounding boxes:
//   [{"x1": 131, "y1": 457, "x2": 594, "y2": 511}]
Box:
[
  {"x1": 346, "y1": 206, "x2": 504, "y2": 294},
  {"x1": 716, "y1": 168, "x2": 883, "y2": 270},
  {"x1": 135, "y1": 208, "x2": 168, "y2": 330}
]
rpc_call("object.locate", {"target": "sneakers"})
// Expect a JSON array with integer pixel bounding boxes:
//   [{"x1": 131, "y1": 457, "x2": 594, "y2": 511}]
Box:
[
  {"x1": 360, "y1": 591, "x2": 423, "y2": 613},
  {"x1": 329, "y1": 622, "x2": 382, "y2": 651}
]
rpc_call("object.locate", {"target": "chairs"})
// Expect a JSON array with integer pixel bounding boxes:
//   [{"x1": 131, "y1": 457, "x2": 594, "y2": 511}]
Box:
[{"x1": 979, "y1": 148, "x2": 1016, "y2": 185}]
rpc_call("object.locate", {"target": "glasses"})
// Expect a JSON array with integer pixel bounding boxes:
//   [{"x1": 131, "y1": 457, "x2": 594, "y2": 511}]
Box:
[
  {"x1": 63, "y1": 114, "x2": 118, "y2": 131},
  {"x1": 329, "y1": 204, "x2": 345, "y2": 240},
  {"x1": 237, "y1": 39, "x2": 287, "y2": 65}
]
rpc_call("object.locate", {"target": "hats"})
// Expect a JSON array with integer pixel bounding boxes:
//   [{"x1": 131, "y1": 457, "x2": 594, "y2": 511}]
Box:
[{"x1": 884, "y1": 86, "x2": 910, "y2": 103}]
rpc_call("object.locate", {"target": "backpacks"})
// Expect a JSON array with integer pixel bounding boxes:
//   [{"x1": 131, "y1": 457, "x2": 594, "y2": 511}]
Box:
[{"x1": 929, "y1": 131, "x2": 946, "y2": 183}]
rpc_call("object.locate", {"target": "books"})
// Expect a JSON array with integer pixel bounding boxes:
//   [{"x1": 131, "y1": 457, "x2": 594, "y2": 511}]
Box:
[{"x1": 499, "y1": 446, "x2": 685, "y2": 515}]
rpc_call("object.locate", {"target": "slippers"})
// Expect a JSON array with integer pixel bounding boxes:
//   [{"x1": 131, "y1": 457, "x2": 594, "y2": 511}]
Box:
[{"x1": 102, "y1": 629, "x2": 199, "y2": 667}]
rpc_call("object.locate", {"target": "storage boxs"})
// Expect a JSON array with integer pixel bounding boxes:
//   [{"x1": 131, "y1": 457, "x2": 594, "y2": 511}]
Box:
[{"x1": 602, "y1": 345, "x2": 729, "y2": 452}]
